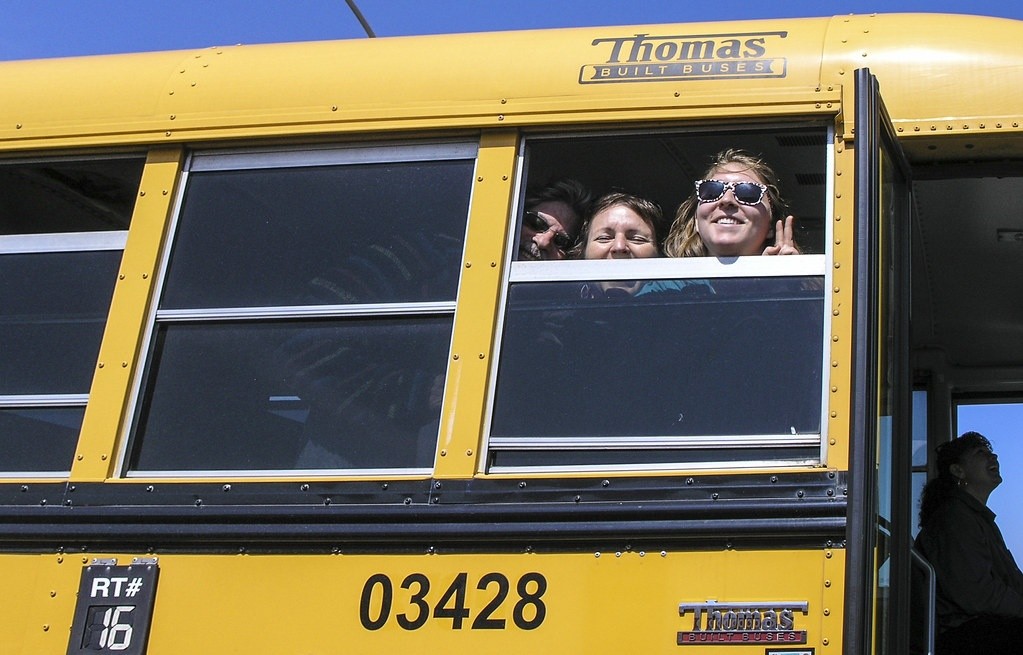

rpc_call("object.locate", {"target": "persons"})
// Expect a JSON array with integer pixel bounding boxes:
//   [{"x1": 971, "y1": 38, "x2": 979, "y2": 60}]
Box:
[
  {"x1": 918, "y1": 432, "x2": 1023, "y2": 655},
  {"x1": 490, "y1": 146, "x2": 823, "y2": 436}
]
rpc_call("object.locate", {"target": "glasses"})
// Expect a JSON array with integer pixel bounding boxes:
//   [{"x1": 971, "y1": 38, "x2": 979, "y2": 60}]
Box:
[
  {"x1": 522, "y1": 210, "x2": 576, "y2": 254},
  {"x1": 695, "y1": 179, "x2": 772, "y2": 207}
]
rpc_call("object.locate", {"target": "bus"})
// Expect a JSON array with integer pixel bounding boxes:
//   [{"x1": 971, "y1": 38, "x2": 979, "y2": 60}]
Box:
[{"x1": 0, "y1": 8, "x2": 1023, "y2": 655}]
